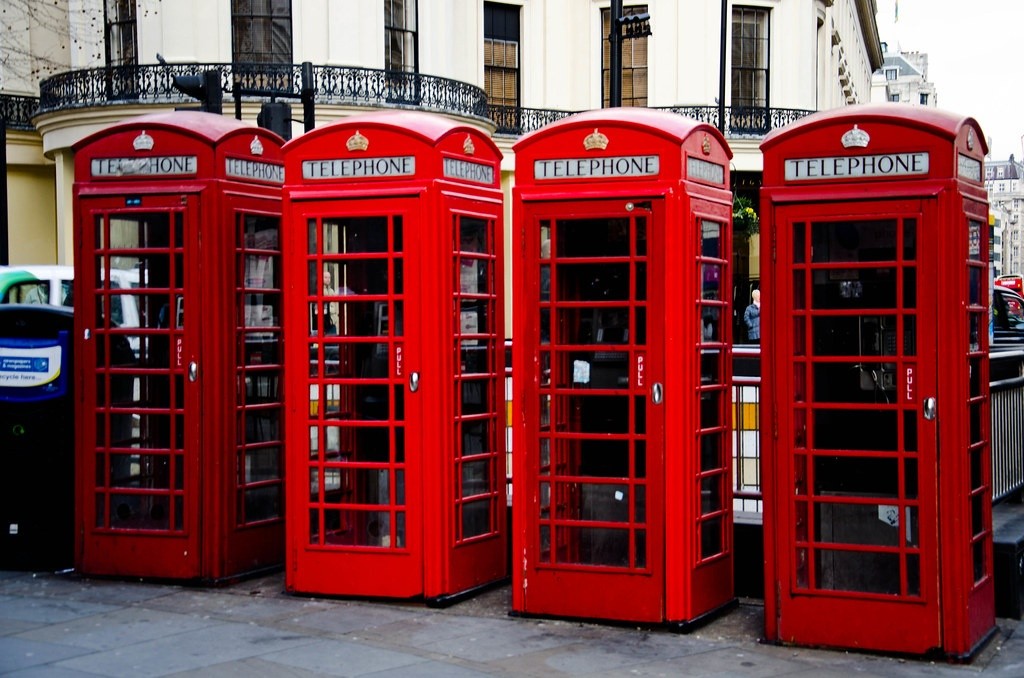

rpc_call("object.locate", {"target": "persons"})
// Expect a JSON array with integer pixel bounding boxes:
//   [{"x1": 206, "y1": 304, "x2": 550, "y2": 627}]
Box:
[
  {"x1": 539, "y1": 238, "x2": 551, "y2": 344},
  {"x1": 25, "y1": 280, "x2": 69, "y2": 307},
  {"x1": 322, "y1": 271, "x2": 339, "y2": 336},
  {"x1": 744, "y1": 290, "x2": 761, "y2": 343}
]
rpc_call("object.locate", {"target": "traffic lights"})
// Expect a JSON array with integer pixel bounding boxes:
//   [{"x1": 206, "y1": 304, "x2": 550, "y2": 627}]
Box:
[{"x1": 173, "y1": 74, "x2": 207, "y2": 101}]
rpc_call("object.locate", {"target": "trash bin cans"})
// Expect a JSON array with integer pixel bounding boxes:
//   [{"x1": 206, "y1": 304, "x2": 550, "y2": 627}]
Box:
[{"x1": 0, "y1": 304, "x2": 137, "y2": 572}]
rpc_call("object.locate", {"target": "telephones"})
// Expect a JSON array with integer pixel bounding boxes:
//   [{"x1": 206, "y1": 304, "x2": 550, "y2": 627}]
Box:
[
  {"x1": 358, "y1": 293, "x2": 402, "y2": 384},
  {"x1": 855, "y1": 300, "x2": 915, "y2": 394},
  {"x1": 575, "y1": 287, "x2": 630, "y2": 367},
  {"x1": 148, "y1": 295, "x2": 184, "y2": 372}
]
